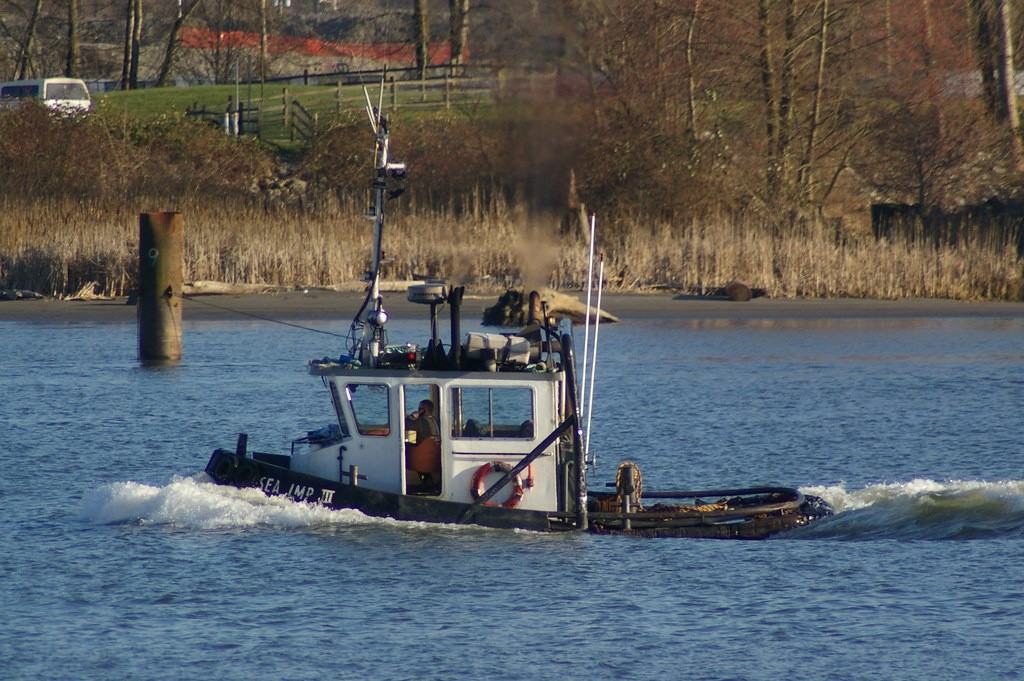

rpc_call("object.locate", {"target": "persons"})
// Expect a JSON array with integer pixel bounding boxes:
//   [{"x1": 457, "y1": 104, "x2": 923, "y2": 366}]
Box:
[{"x1": 405, "y1": 399, "x2": 441, "y2": 492}]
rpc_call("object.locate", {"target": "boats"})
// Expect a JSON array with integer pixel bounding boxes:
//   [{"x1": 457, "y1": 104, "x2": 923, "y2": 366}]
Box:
[{"x1": 204, "y1": 64, "x2": 835, "y2": 539}]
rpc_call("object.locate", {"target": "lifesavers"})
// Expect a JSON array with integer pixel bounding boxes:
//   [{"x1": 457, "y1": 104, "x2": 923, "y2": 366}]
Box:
[{"x1": 470, "y1": 460, "x2": 524, "y2": 508}]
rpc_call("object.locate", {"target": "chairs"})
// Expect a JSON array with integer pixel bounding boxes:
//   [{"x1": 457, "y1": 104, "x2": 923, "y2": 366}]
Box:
[{"x1": 404, "y1": 435, "x2": 441, "y2": 494}]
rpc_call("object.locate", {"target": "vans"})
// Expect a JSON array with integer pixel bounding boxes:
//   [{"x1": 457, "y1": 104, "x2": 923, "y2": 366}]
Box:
[{"x1": 0, "y1": 77, "x2": 92, "y2": 121}]
